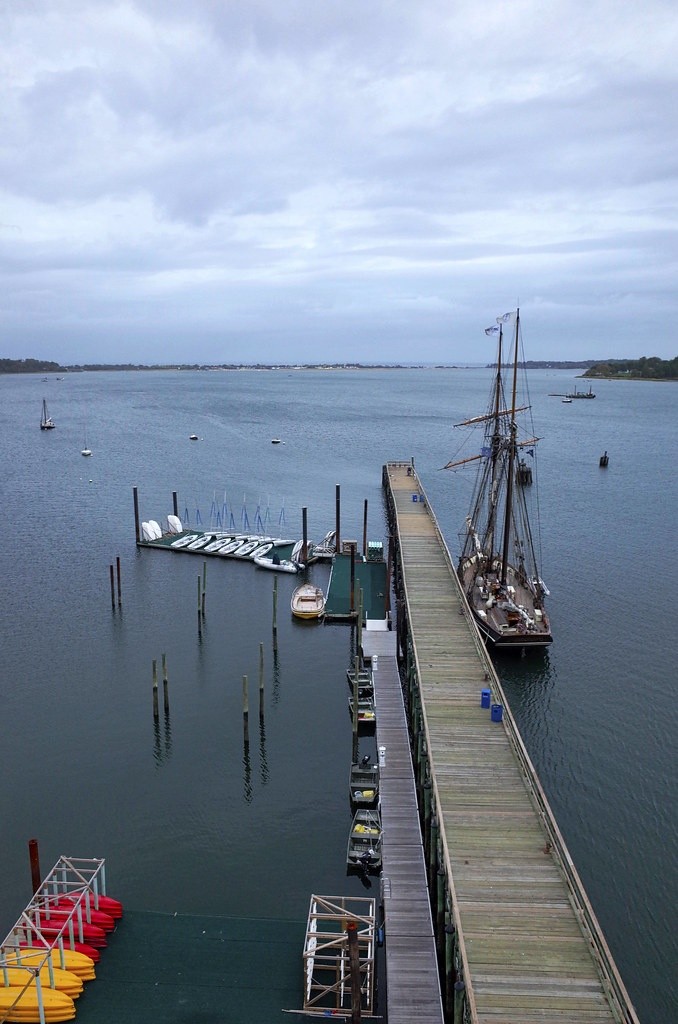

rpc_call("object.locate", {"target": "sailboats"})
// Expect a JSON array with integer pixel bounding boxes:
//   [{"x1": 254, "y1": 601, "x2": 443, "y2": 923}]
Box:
[
  {"x1": 440, "y1": 304, "x2": 556, "y2": 652},
  {"x1": 37, "y1": 397, "x2": 54, "y2": 430}
]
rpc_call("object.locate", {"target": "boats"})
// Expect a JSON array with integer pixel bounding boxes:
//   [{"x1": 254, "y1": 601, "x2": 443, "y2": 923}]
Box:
[
  {"x1": 349, "y1": 755, "x2": 379, "y2": 803},
  {"x1": 253, "y1": 555, "x2": 305, "y2": 576},
  {"x1": 56, "y1": 376, "x2": 64, "y2": 381},
  {"x1": 346, "y1": 667, "x2": 375, "y2": 693},
  {"x1": 566, "y1": 385, "x2": 595, "y2": 399},
  {"x1": 234, "y1": 540, "x2": 258, "y2": 557},
  {"x1": 348, "y1": 696, "x2": 375, "y2": 725},
  {"x1": 204, "y1": 537, "x2": 232, "y2": 553},
  {"x1": 249, "y1": 543, "x2": 273, "y2": 559},
  {"x1": 218, "y1": 540, "x2": 244, "y2": 556},
  {"x1": 40, "y1": 375, "x2": 49, "y2": 382},
  {"x1": 0, "y1": 893, "x2": 124, "y2": 1024},
  {"x1": 309, "y1": 530, "x2": 338, "y2": 558},
  {"x1": 188, "y1": 535, "x2": 212, "y2": 553},
  {"x1": 345, "y1": 809, "x2": 385, "y2": 868},
  {"x1": 515, "y1": 459, "x2": 533, "y2": 487},
  {"x1": 599, "y1": 448, "x2": 609, "y2": 467},
  {"x1": 290, "y1": 581, "x2": 325, "y2": 619},
  {"x1": 169, "y1": 534, "x2": 199, "y2": 550}
]
